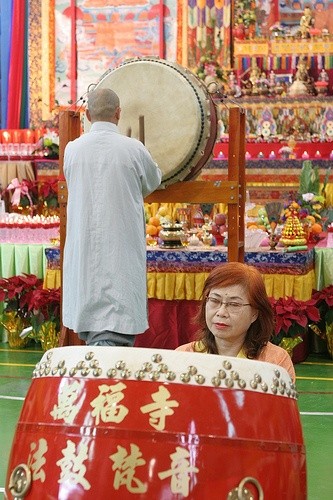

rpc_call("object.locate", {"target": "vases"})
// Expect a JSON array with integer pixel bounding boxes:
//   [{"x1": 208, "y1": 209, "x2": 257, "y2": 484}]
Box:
[{"x1": 284, "y1": 334, "x2": 307, "y2": 364}]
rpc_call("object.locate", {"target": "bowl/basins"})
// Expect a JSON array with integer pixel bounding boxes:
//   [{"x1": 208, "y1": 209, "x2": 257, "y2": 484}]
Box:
[{"x1": 222, "y1": 229, "x2": 268, "y2": 251}]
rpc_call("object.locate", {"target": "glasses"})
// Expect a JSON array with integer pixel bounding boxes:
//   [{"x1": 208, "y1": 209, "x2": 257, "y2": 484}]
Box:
[{"x1": 204, "y1": 295, "x2": 250, "y2": 313}]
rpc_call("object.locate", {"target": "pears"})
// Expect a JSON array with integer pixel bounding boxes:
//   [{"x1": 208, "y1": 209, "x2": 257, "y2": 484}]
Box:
[{"x1": 155, "y1": 207, "x2": 172, "y2": 222}]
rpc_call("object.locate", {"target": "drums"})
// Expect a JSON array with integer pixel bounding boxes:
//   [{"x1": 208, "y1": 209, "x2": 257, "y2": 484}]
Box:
[
  {"x1": 4, "y1": 349, "x2": 306, "y2": 500},
  {"x1": 84, "y1": 60, "x2": 218, "y2": 191}
]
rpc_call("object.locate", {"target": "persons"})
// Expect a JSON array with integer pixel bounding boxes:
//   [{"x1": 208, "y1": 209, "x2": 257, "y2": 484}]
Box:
[
  {"x1": 61, "y1": 87, "x2": 160, "y2": 347},
  {"x1": 300, "y1": 6, "x2": 315, "y2": 38},
  {"x1": 173, "y1": 261, "x2": 295, "y2": 384}
]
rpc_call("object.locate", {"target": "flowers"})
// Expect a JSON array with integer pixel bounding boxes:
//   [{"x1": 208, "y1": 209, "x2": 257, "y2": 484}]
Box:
[
  {"x1": 264, "y1": 285, "x2": 333, "y2": 345},
  {"x1": 6, "y1": 173, "x2": 58, "y2": 215},
  {"x1": 37, "y1": 130, "x2": 61, "y2": 160},
  {"x1": 0, "y1": 272, "x2": 62, "y2": 327},
  {"x1": 256, "y1": 159, "x2": 333, "y2": 252}
]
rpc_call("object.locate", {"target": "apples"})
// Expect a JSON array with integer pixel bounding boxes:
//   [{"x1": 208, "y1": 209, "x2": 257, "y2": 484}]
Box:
[{"x1": 211, "y1": 213, "x2": 228, "y2": 235}]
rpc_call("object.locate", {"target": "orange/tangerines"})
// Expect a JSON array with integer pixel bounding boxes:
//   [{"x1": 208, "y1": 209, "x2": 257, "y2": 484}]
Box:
[
  {"x1": 247, "y1": 222, "x2": 266, "y2": 232},
  {"x1": 146, "y1": 217, "x2": 163, "y2": 236},
  {"x1": 307, "y1": 216, "x2": 322, "y2": 233}
]
